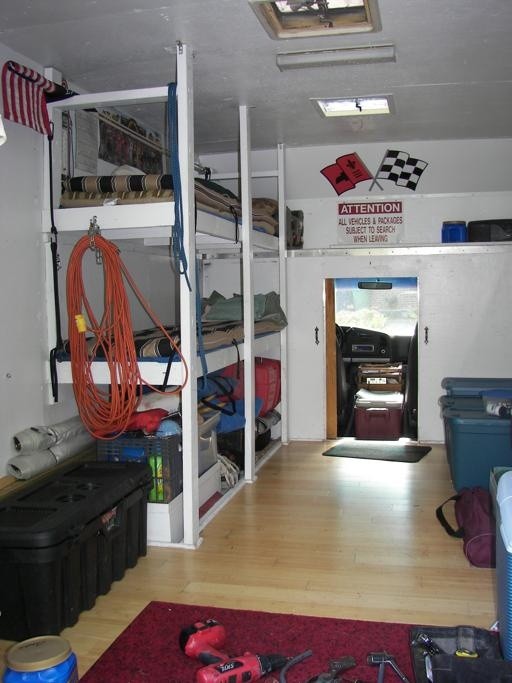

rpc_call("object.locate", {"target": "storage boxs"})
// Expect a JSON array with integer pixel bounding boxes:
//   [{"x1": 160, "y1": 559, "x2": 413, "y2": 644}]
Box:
[
  {"x1": 0, "y1": 461, "x2": 154, "y2": 642},
  {"x1": 353, "y1": 390, "x2": 404, "y2": 441},
  {"x1": 437, "y1": 377, "x2": 512, "y2": 493}
]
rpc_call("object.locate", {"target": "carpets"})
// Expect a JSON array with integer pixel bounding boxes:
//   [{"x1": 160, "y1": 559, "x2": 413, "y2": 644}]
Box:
[
  {"x1": 75, "y1": 600, "x2": 504, "y2": 683},
  {"x1": 322, "y1": 439, "x2": 432, "y2": 463}
]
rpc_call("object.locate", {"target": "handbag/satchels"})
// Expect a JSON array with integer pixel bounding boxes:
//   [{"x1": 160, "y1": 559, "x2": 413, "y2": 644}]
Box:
[{"x1": 436, "y1": 486, "x2": 496, "y2": 568}]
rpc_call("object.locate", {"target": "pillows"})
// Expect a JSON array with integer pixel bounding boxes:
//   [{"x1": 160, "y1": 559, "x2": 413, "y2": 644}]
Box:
[
  {"x1": 239, "y1": 197, "x2": 280, "y2": 217},
  {"x1": 198, "y1": 287, "x2": 281, "y2": 322}
]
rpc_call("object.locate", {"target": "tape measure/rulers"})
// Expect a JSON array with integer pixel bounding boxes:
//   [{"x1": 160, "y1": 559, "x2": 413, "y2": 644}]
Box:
[{"x1": 455, "y1": 646, "x2": 478, "y2": 658}]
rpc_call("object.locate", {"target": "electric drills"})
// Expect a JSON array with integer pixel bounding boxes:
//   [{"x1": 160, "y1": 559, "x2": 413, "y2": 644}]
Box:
[{"x1": 179, "y1": 620, "x2": 294, "y2": 683}]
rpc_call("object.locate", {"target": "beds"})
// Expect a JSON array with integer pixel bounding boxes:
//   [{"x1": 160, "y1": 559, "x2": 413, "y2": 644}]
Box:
[{"x1": 38, "y1": 42, "x2": 287, "y2": 410}]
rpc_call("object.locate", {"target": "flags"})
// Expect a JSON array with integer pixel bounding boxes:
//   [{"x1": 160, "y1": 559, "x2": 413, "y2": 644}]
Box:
[
  {"x1": 320, "y1": 152, "x2": 373, "y2": 196},
  {"x1": 377, "y1": 150, "x2": 428, "y2": 192}
]
